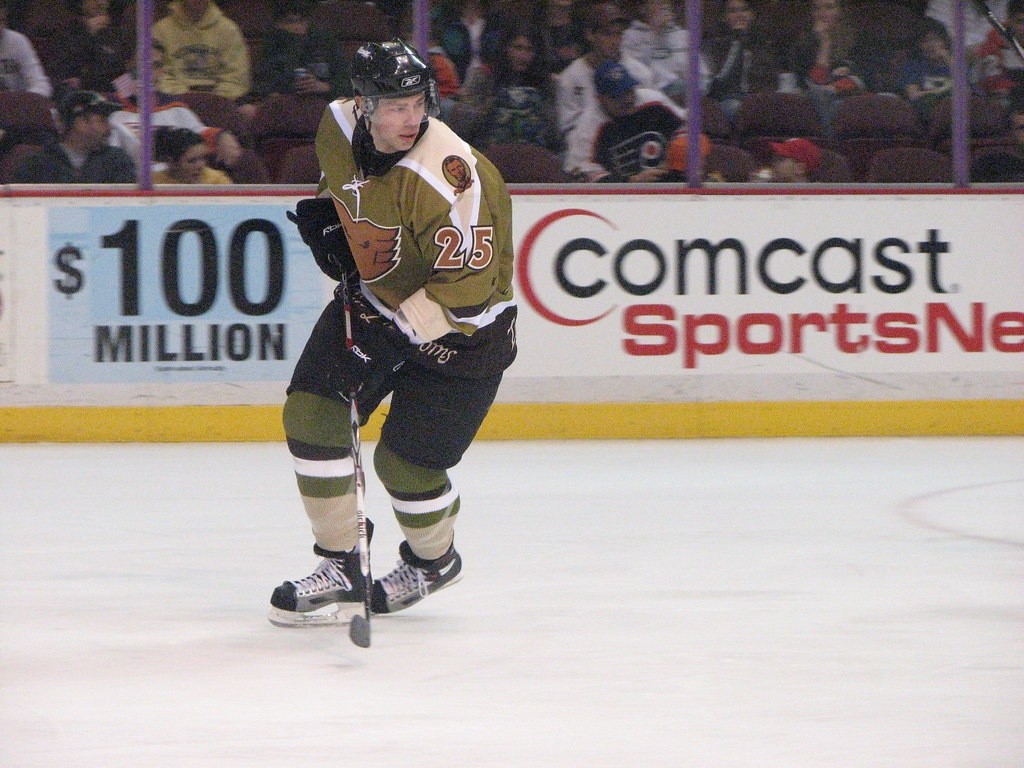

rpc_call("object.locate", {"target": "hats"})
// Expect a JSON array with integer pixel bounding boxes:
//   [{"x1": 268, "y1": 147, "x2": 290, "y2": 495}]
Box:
[
  {"x1": 593, "y1": 62, "x2": 641, "y2": 97},
  {"x1": 584, "y1": 3, "x2": 630, "y2": 31},
  {"x1": 58, "y1": 90, "x2": 123, "y2": 125}
]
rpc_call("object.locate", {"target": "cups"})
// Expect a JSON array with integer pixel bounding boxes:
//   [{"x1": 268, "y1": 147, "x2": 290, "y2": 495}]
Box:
[{"x1": 779, "y1": 72, "x2": 798, "y2": 91}]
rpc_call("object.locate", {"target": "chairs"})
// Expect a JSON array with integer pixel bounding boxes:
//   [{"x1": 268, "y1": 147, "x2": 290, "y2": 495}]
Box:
[
  {"x1": 179, "y1": 90, "x2": 565, "y2": 183},
  {"x1": 670, "y1": 87, "x2": 1024, "y2": 186}
]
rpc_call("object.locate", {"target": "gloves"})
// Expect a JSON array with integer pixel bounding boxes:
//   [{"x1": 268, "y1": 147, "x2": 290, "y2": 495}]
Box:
[
  {"x1": 335, "y1": 320, "x2": 411, "y2": 427},
  {"x1": 285, "y1": 198, "x2": 360, "y2": 287}
]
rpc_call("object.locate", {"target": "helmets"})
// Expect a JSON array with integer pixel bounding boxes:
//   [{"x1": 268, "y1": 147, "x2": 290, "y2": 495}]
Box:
[{"x1": 349, "y1": 37, "x2": 439, "y2": 118}]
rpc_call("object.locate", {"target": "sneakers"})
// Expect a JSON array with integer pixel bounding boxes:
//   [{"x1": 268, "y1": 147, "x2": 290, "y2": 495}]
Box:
[
  {"x1": 369, "y1": 530, "x2": 463, "y2": 616},
  {"x1": 266, "y1": 518, "x2": 374, "y2": 625}
]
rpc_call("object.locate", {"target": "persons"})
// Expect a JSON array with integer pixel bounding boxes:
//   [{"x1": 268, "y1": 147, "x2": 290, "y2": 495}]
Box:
[
  {"x1": 268, "y1": 36, "x2": 518, "y2": 626},
  {"x1": 0, "y1": 0, "x2": 1024, "y2": 186}
]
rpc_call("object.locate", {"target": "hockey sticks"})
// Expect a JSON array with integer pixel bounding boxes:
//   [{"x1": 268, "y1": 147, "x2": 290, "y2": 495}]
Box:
[{"x1": 341, "y1": 272, "x2": 373, "y2": 650}]
